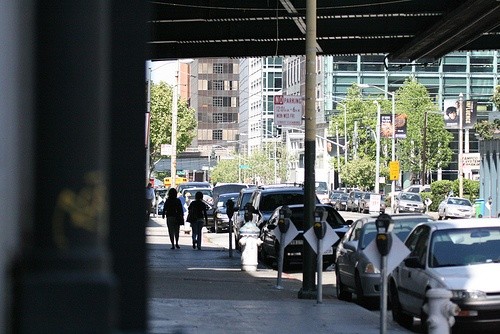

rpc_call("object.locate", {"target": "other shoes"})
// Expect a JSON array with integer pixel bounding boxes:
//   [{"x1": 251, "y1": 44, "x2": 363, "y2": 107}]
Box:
[
  {"x1": 192, "y1": 245, "x2": 196, "y2": 249},
  {"x1": 197, "y1": 245, "x2": 201, "y2": 251},
  {"x1": 170, "y1": 247, "x2": 175, "y2": 250},
  {"x1": 175, "y1": 244, "x2": 180, "y2": 249}
]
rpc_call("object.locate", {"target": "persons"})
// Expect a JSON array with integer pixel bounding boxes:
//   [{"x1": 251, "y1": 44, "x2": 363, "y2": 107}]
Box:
[
  {"x1": 162, "y1": 188, "x2": 184, "y2": 249},
  {"x1": 178, "y1": 192, "x2": 191, "y2": 234},
  {"x1": 145, "y1": 183, "x2": 155, "y2": 221},
  {"x1": 188, "y1": 191, "x2": 208, "y2": 250},
  {"x1": 445, "y1": 107, "x2": 459, "y2": 124}
]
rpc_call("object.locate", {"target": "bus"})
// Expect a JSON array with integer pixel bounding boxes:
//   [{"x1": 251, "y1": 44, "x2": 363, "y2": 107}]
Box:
[{"x1": 294, "y1": 181, "x2": 330, "y2": 204}]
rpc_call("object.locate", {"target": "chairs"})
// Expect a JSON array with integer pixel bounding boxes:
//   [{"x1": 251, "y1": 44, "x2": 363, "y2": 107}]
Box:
[
  {"x1": 398, "y1": 231, "x2": 410, "y2": 242},
  {"x1": 364, "y1": 231, "x2": 377, "y2": 246},
  {"x1": 433, "y1": 240, "x2": 464, "y2": 266}
]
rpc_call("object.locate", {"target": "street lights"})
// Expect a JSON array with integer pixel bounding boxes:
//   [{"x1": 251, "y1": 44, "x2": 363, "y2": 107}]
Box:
[
  {"x1": 357, "y1": 83, "x2": 396, "y2": 209},
  {"x1": 145, "y1": 58, "x2": 195, "y2": 187},
  {"x1": 256, "y1": 127, "x2": 277, "y2": 185},
  {"x1": 315, "y1": 97, "x2": 347, "y2": 191},
  {"x1": 226, "y1": 140, "x2": 246, "y2": 182}
]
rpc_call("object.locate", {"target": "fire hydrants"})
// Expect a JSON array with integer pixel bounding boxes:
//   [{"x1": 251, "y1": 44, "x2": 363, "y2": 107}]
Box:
[
  {"x1": 239, "y1": 222, "x2": 264, "y2": 273},
  {"x1": 422, "y1": 287, "x2": 461, "y2": 334}
]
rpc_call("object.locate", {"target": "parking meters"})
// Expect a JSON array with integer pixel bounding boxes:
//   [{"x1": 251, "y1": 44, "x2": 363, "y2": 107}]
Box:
[
  {"x1": 312, "y1": 205, "x2": 329, "y2": 303},
  {"x1": 274, "y1": 204, "x2": 293, "y2": 288},
  {"x1": 225, "y1": 198, "x2": 235, "y2": 257},
  {"x1": 243, "y1": 202, "x2": 253, "y2": 223},
  {"x1": 374, "y1": 210, "x2": 393, "y2": 334}
]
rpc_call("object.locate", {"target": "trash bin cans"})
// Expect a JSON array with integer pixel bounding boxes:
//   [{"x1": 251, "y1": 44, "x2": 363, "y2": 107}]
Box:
[{"x1": 475, "y1": 199, "x2": 484, "y2": 218}]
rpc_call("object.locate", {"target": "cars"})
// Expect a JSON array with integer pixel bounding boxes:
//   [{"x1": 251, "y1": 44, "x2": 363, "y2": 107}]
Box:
[
  {"x1": 334, "y1": 211, "x2": 437, "y2": 309},
  {"x1": 388, "y1": 217, "x2": 500, "y2": 334},
  {"x1": 386, "y1": 184, "x2": 432, "y2": 214},
  {"x1": 258, "y1": 203, "x2": 355, "y2": 270},
  {"x1": 438, "y1": 197, "x2": 476, "y2": 221},
  {"x1": 154, "y1": 181, "x2": 322, "y2": 252},
  {"x1": 329, "y1": 187, "x2": 388, "y2": 215}
]
